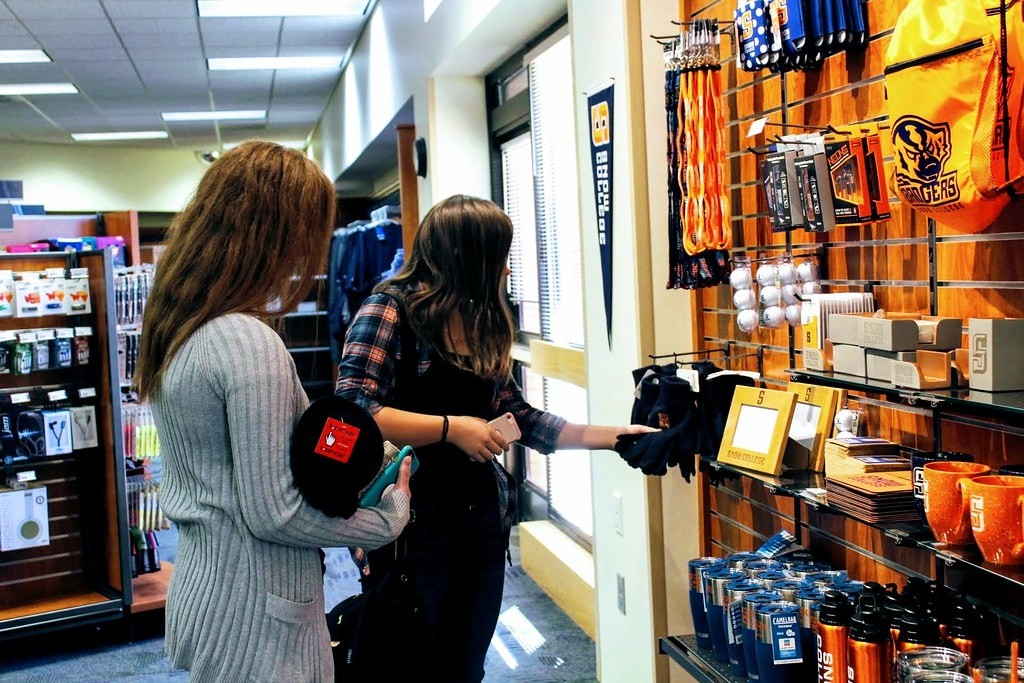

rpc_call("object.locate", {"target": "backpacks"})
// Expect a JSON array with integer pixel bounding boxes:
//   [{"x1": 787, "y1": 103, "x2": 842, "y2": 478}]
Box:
[{"x1": 882, "y1": 0, "x2": 1024, "y2": 234}]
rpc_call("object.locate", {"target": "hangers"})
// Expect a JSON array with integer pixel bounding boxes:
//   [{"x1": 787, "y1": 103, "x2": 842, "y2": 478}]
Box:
[{"x1": 333, "y1": 205, "x2": 401, "y2": 237}]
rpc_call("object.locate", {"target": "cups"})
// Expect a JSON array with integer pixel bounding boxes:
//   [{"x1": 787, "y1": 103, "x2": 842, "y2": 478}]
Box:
[
  {"x1": 725, "y1": 551, "x2": 761, "y2": 571},
  {"x1": 742, "y1": 593, "x2": 782, "y2": 683},
  {"x1": 770, "y1": 580, "x2": 809, "y2": 602},
  {"x1": 970, "y1": 475, "x2": 1024, "y2": 565},
  {"x1": 754, "y1": 601, "x2": 800, "y2": 683},
  {"x1": 795, "y1": 591, "x2": 823, "y2": 629},
  {"x1": 776, "y1": 557, "x2": 865, "y2": 605},
  {"x1": 912, "y1": 452, "x2": 974, "y2": 527},
  {"x1": 923, "y1": 461, "x2": 990, "y2": 545},
  {"x1": 999, "y1": 464, "x2": 1024, "y2": 478},
  {"x1": 722, "y1": 579, "x2": 759, "y2": 677},
  {"x1": 757, "y1": 572, "x2": 787, "y2": 590},
  {"x1": 706, "y1": 568, "x2": 742, "y2": 663},
  {"x1": 810, "y1": 603, "x2": 821, "y2": 635},
  {"x1": 741, "y1": 560, "x2": 783, "y2": 579},
  {"x1": 688, "y1": 557, "x2": 727, "y2": 648}
]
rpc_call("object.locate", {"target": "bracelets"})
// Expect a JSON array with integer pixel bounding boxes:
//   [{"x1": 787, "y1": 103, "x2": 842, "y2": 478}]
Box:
[{"x1": 440, "y1": 415, "x2": 449, "y2": 442}]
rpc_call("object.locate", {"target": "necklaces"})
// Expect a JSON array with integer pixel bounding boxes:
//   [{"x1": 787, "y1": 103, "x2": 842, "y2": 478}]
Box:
[{"x1": 446, "y1": 320, "x2": 466, "y2": 370}]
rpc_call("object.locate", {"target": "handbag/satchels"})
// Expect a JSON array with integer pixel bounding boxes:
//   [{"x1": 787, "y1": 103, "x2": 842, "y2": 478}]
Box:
[{"x1": 371, "y1": 507, "x2": 430, "y2": 581}]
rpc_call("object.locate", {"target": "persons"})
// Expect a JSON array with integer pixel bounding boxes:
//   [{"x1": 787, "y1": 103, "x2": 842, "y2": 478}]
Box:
[
  {"x1": 130, "y1": 140, "x2": 412, "y2": 683},
  {"x1": 333, "y1": 194, "x2": 662, "y2": 683}
]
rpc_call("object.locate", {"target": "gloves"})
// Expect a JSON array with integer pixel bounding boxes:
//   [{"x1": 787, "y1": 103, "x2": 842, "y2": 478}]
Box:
[{"x1": 610, "y1": 361, "x2": 756, "y2": 483}]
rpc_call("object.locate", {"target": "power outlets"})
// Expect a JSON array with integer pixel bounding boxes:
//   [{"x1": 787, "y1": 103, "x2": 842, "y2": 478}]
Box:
[{"x1": 616, "y1": 572, "x2": 626, "y2": 615}]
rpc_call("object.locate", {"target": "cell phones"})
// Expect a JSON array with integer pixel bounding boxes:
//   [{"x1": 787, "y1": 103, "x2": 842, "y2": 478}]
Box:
[
  {"x1": 470, "y1": 412, "x2": 521, "y2": 461},
  {"x1": 360, "y1": 445, "x2": 419, "y2": 509}
]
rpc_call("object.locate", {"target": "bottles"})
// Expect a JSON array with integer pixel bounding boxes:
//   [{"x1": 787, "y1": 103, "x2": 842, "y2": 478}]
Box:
[
  {"x1": 854, "y1": 577, "x2": 980, "y2": 683},
  {"x1": 897, "y1": 646, "x2": 968, "y2": 683},
  {"x1": 817, "y1": 590, "x2": 847, "y2": 683},
  {"x1": 846, "y1": 593, "x2": 884, "y2": 683},
  {"x1": 975, "y1": 656, "x2": 1024, "y2": 683},
  {"x1": 904, "y1": 670, "x2": 974, "y2": 683}
]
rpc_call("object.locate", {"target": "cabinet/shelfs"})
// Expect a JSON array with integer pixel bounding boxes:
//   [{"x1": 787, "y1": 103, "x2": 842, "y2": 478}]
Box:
[
  {"x1": 0, "y1": 195, "x2": 375, "y2": 641},
  {"x1": 649, "y1": 1, "x2": 1024, "y2": 683}
]
